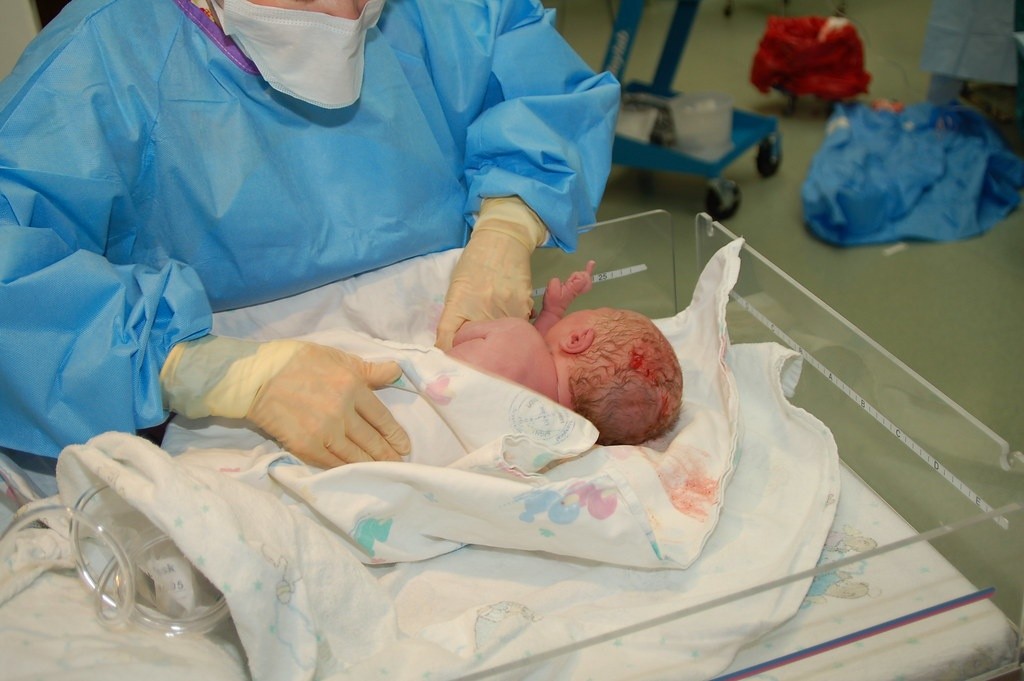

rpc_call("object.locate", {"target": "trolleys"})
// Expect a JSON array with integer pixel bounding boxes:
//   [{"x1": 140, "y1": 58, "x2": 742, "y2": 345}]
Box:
[{"x1": 600, "y1": 2, "x2": 787, "y2": 222}]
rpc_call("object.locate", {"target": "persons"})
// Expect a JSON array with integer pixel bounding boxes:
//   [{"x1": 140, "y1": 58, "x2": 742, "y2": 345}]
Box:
[
  {"x1": 0, "y1": 0, "x2": 620, "y2": 471},
  {"x1": 451, "y1": 259, "x2": 684, "y2": 445}
]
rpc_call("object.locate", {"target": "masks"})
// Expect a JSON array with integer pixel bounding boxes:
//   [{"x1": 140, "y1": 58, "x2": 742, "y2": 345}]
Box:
[{"x1": 211, "y1": 0, "x2": 385, "y2": 110}]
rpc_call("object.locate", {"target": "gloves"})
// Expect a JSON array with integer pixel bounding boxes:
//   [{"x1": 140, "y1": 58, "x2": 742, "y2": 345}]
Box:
[
  {"x1": 155, "y1": 332, "x2": 410, "y2": 466},
  {"x1": 436, "y1": 194, "x2": 550, "y2": 353}
]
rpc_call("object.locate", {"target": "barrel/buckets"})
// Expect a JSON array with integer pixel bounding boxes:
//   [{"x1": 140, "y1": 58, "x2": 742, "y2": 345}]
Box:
[{"x1": 671, "y1": 92, "x2": 734, "y2": 163}]
